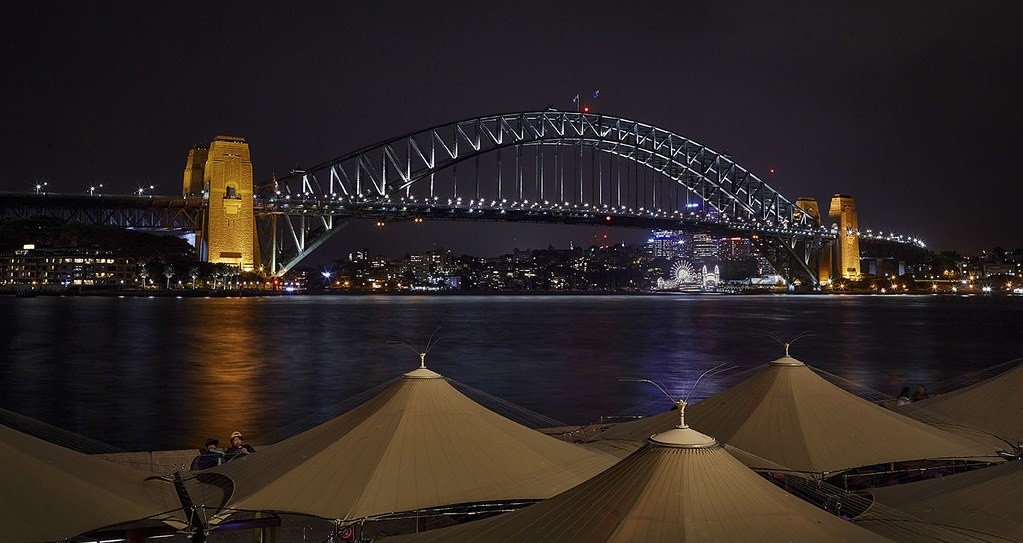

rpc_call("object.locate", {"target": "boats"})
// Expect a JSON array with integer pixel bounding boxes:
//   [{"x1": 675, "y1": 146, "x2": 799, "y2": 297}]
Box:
[{"x1": 16, "y1": 289, "x2": 37, "y2": 298}]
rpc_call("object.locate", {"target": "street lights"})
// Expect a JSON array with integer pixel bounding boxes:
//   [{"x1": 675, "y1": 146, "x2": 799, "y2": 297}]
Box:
[
  {"x1": 139, "y1": 189, "x2": 142, "y2": 195},
  {"x1": 91, "y1": 188, "x2": 94, "y2": 196},
  {"x1": 36, "y1": 185, "x2": 40, "y2": 195},
  {"x1": 604, "y1": 234, "x2": 607, "y2": 248}
]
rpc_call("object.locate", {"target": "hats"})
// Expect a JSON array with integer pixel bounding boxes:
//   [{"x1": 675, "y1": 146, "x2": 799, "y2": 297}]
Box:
[{"x1": 205, "y1": 438, "x2": 219, "y2": 447}]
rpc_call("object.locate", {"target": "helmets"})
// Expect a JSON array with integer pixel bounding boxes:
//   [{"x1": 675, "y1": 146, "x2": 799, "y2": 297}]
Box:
[{"x1": 230, "y1": 431, "x2": 243, "y2": 439}]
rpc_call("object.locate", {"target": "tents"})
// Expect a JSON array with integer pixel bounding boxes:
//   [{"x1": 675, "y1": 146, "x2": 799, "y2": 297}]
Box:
[{"x1": 0, "y1": 344, "x2": 1022, "y2": 543}]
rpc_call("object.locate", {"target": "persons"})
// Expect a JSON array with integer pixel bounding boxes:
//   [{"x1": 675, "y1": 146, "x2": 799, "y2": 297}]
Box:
[
  {"x1": 887, "y1": 475, "x2": 897, "y2": 485},
  {"x1": 897, "y1": 388, "x2": 911, "y2": 408},
  {"x1": 912, "y1": 382, "x2": 928, "y2": 400},
  {"x1": 883, "y1": 383, "x2": 895, "y2": 401},
  {"x1": 841, "y1": 512, "x2": 850, "y2": 522},
  {"x1": 223, "y1": 431, "x2": 256, "y2": 462},
  {"x1": 191, "y1": 437, "x2": 223, "y2": 470}
]
